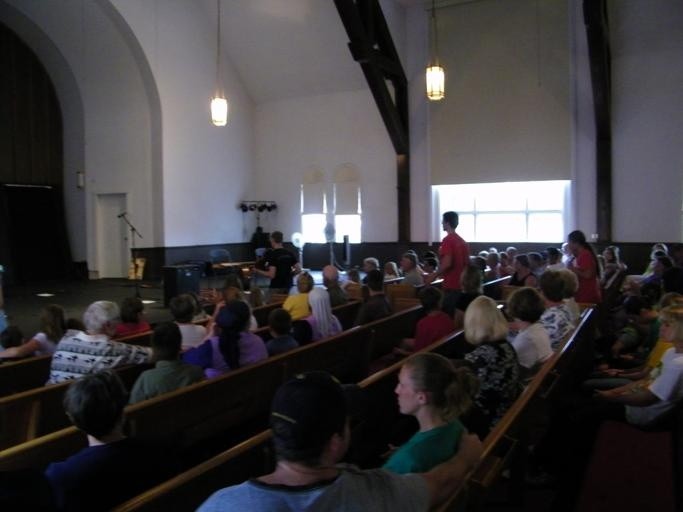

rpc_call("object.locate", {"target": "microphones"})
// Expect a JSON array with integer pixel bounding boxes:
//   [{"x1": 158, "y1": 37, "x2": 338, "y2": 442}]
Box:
[{"x1": 118, "y1": 212, "x2": 127, "y2": 218}]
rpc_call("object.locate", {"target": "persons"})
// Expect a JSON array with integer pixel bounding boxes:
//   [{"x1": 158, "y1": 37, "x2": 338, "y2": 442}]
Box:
[
  {"x1": 252, "y1": 231, "x2": 301, "y2": 289},
  {"x1": 252, "y1": 227, "x2": 267, "y2": 261},
  {"x1": 0, "y1": 244, "x2": 682, "y2": 511},
  {"x1": 568, "y1": 231, "x2": 602, "y2": 303},
  {"x1": 426, "y1": 212, "x2": 469, "y2": 316}
]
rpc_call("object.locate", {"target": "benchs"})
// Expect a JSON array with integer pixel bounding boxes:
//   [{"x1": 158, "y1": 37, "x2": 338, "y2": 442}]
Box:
[{"x1": 0, "y1": 251, "x2": 682, "y2": 510}]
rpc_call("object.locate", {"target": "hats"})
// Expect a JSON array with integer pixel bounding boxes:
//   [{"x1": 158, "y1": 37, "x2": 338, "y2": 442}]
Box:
[{"x1": 268, "y1": 370, "x2": 365, "y2": 442}]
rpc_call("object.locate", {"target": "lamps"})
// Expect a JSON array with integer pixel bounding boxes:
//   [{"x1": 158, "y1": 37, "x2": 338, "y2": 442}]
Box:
[
  {"x1": 200, "y1": 2, "x2": 239, "y2": 129},
  {"x1": 418, "y1": 1, "x2": 448, "y2": 103}
]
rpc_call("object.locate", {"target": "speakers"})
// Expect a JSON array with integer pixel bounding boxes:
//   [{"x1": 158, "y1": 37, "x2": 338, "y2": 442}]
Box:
[{"x1": 162, "y1": 264, "x2": 201, "y2": 308}]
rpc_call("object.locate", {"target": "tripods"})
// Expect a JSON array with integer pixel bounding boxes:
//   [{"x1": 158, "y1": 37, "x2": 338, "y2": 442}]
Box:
[{"x1": 126, "y1": 228, "x2": 161, "y2": 300}]
